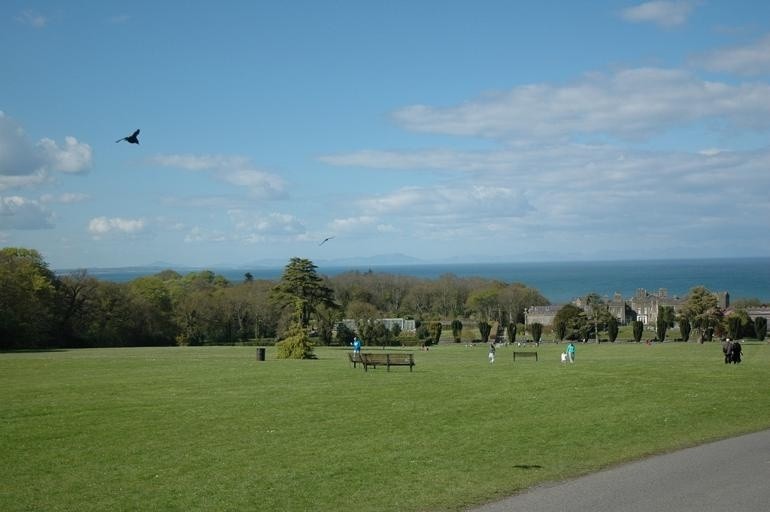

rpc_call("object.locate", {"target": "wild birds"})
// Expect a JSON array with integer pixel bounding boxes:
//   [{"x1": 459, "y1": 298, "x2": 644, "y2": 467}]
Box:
[
  {"x1": 320, "y1": 235, "x2": 336, "y2": 245},
  {"x1": 116, "y1": 129, "x2": 142, "y2": 145}
]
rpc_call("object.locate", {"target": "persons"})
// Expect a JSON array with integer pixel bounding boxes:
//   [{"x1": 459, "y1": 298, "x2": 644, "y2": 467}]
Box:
[
  {"x1": 489, "y1": 346, "x2": 495, "y2": 364},
  {"x1": 560, "y1": 352, "x2": 568, "y2": 365},
  {"x1": 722, "y1": 339, "x2": 733, "y2": 364},
  {"x1": 350, "y1": 337, "x2": 361, "y2": 358},
  {"x1": 566, "y1": 342, "x2": 576, "y2": 364},
  {"x1": 732, "y1": 340, "x2": 741, "y2": 364}
]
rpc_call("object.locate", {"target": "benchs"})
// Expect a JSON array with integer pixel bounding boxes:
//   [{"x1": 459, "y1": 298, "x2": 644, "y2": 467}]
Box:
[
  {"x1": 362, "y1": 352, "x2": 415, "y2": 372},
  {"x1": 348, "y1": 350, "x2": 377, "y2": 368},
  {"x1": 512, "y1": 351, "x2": 539, "y2": 362}
]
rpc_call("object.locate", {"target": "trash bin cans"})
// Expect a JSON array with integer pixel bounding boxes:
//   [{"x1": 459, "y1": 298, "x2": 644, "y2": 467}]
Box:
[{"x1": 256, "y1": 348, "x2": 265, "y2": 361}]
